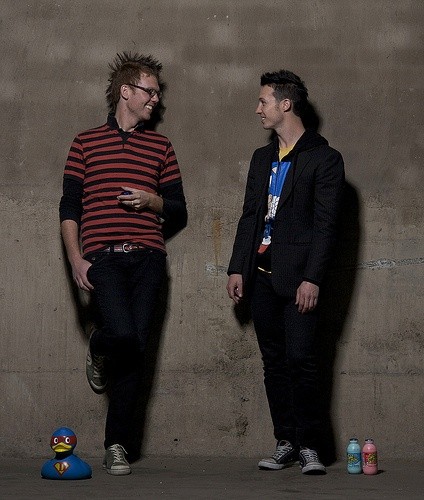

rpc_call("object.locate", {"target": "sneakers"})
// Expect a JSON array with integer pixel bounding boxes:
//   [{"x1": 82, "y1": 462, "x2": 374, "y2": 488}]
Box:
[
  {"x1": 86, "y1": 327, "x2": 110, "y2": 390},
  {"x1": 258, "y1": 440, "x2": 297, "y2": 470},
  {"x1": 297, "y1": 445, "x2": 326, "y2": 473},
  {"x1": 102, "y1": 444, "x2": 131, "y2": 475}
]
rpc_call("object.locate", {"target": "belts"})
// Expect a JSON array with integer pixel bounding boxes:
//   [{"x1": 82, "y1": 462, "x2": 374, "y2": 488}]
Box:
[{"x1": 104, "y1": 243, "x2": 147, "y2": 253}]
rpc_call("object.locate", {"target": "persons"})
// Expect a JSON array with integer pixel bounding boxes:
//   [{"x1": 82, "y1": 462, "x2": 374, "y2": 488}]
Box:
[
  {"x1": 57, "y1": 49, "x2": 188, "y2": 476},
  {"x1": 224, "y1": 68, "x2": 346, "y2": 475}
]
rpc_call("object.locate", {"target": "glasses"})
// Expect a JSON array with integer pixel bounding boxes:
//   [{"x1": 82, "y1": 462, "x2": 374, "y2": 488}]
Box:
[{"x1": 124, "y1": 83, "x2": 163, "y2": 99}]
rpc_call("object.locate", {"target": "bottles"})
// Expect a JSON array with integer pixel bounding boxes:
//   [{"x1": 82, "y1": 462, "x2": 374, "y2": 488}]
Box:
[
  {"x1": 346, "y1": 437, "x2": 362, "y2": 474},
  {"x1": 363, "y1": 438, "x2": 379, "y2": 476}
]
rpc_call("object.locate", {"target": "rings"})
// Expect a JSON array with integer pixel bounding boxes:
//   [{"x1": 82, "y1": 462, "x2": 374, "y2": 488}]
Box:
[{"x1": 131, "y1": 201, "x2": 134, "y2": 206}]
